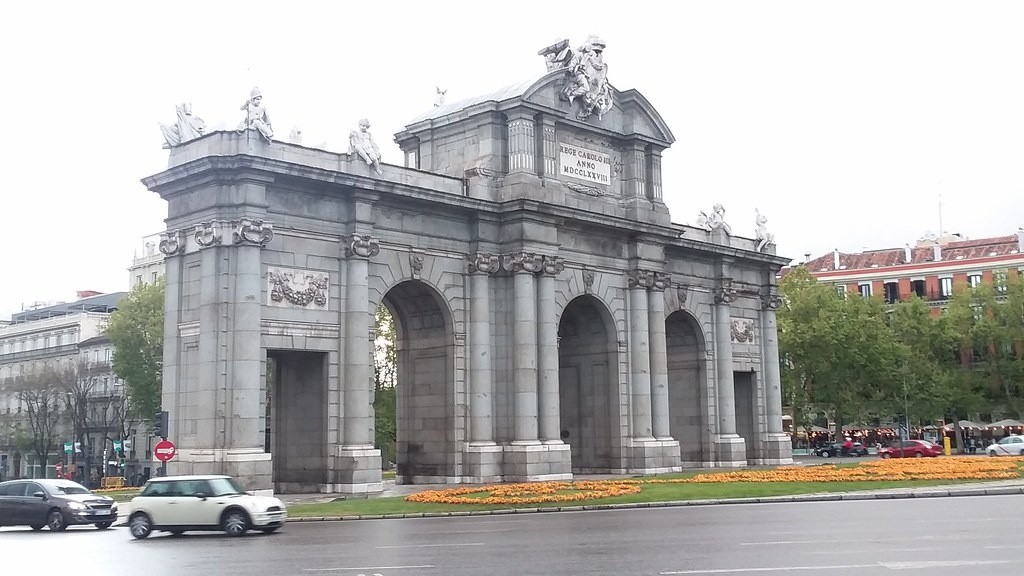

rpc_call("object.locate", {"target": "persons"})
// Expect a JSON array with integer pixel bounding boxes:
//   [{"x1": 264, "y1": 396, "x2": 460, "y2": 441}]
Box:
[
  {"x1": 351, "y1": 118, "x2": 382, "y2": 175},
  {"x1": 434, "y1": 86, "x2": 448, "y2": 111},
  {"x1": 568, "y1": 42, "x2": 603, "y2": 106},
  {"x1": 756, "y1": 210, "x2": 772, "y2": 252},
  {"x1": 708, "y1": 204, "x2": 731, "y2": 237},
  {"x1": 786, "y1": 425, "x2": 996, "y2": 457},
  {"x1": 239, "y1": 87, "x2": 273, "y2": 145}
]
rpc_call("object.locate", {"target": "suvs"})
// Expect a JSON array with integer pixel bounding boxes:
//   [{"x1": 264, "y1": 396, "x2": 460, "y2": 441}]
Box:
[{"x1": 128, "y1": 475, "x2": 289, "y2": 539}]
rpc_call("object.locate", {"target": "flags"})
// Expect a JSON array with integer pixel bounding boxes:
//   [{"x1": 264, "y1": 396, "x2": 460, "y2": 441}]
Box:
[
  {"x1": 74, "y1": 441, "x2": 82, "y2": 453},
  {"x1": 113, "y1": 439, "x2": 121, "y2": 452},
  {"x1": 123, "y1": 439, "x2": 131, "y2": 451},
  {"x1": 934, "y1": 185, "x2": 951, "y2": 212},
  {"x1": 64, "y1": 442, "x2": 73, "y2": 453}
]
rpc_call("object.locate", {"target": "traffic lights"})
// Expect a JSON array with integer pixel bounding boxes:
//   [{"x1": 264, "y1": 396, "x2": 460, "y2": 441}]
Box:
[{"x1": 153, "y1": 411, "x2": 168, "y2": 438}]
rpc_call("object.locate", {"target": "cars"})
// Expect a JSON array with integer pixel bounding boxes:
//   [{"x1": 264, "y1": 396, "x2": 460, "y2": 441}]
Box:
[
  {"x1": 880, "y1": 440, "x2": 944, "y2": 458},
  {"x1": 985, "y1": 434, "x2": 1024, "y2": 456},
  {"x1": 0, "y1": 478, "x2": 118, "y2": 532},
  {"x1": 817, "y1": 442, "x2": 868, "y2": 458}
]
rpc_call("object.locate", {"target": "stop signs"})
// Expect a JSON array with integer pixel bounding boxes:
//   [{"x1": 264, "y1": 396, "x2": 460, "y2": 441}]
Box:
[{"x1": 155, "y1": 440, "x2": 175, "y2": 461}]
